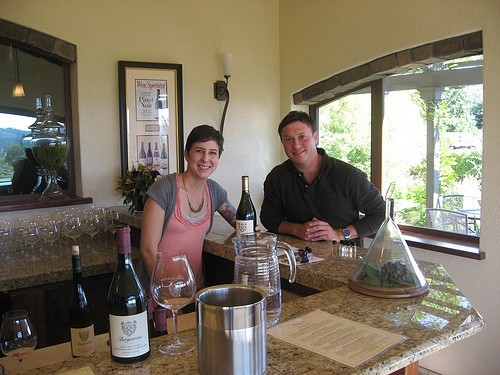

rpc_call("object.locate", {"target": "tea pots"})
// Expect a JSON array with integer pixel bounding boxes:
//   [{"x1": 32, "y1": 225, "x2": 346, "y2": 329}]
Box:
[{"x1": 231, "y1": 231, "x2": 297, "y2": 328}]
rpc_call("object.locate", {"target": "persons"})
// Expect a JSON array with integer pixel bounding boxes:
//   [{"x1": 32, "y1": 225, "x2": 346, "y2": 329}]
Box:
[
  {"x1": 139, "y1": 124, "x2": 262, "y2": 317},
  {"x1": 259, "y1": 110, "x2": 386, "y2": 248}
]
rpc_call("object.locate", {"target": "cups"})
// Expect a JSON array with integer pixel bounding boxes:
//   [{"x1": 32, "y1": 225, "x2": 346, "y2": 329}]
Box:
[{"x1": 194, "y1": 283, "x2": 268, "y2": 372}]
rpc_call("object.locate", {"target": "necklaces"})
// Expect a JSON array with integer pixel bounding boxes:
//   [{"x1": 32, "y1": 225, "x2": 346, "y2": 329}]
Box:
[{"x1": 182, "y1": 173, "x2": 205, "y2": 213}]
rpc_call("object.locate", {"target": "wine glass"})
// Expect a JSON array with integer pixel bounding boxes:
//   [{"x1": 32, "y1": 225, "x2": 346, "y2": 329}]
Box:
[
  {"x1": 149, "y1": 249, "x2": 198, "y2": 356},
  {"x1": 0, "y1": 219, "x2": 14, "y2": 256},
  {"x1": 14, "y1": 204, "x2": 122, "y2": 261},
  {"x1": 0, "y1": 310, "x2": 38, "y2": 375}
]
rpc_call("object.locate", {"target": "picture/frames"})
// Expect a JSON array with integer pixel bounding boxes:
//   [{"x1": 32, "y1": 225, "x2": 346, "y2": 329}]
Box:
[{"x1": 119, "y1": 61, "x2": 185, "y2": 179}]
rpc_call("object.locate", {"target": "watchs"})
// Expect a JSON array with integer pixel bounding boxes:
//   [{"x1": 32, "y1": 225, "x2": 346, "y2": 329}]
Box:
[{"x1": 342, "y1": 227, "x2": 351, "y2": 241}]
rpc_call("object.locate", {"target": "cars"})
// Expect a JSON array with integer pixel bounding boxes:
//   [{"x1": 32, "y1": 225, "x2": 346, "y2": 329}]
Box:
[{"x1": 436, "y1": 194, "x2": 481, "y2": 215}]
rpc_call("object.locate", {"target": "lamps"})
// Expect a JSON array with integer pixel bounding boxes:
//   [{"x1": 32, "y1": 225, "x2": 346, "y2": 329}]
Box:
[{"x1": 12, "y1": 45, "x2": 28, "y2": 97}]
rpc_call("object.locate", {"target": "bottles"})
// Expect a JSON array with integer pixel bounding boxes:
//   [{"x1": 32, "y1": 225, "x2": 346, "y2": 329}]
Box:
[
  {"x1": 153, "y1": 142, "x2": 160, "y2": 166},
  {"x1": 235, "y1": 176, "x2": 256, "y2": 254},
  {"x1": 152, "y1": 306, "x2": 170, "y2": 337},
  {"x1": 154, "y1": 88, "x2": 163, "y2": 119},
  {"x1": 146, "y1": 142, "x2": 153, "y2": 166},
  {"x1": 106, "y1": 223, "x2": 151, "y2": 363},
  {"x1": 139, "y1": 141, "x2": 147, "y2": 167},
  {"x1": 147, "y1": 295, "x2": 155, "y2": 339},
  {"x1": 68, "y1": 244, "x2": 96, "y2": 358},
  {"x1": 160, "y1": 143, "x2": 169, "y2": 169}
]
